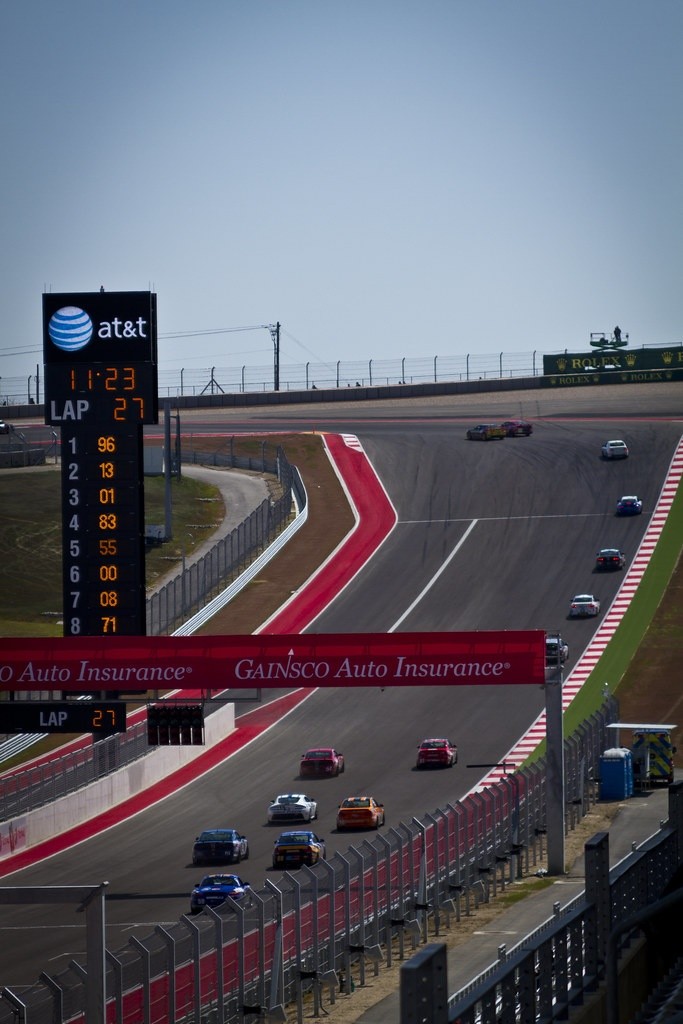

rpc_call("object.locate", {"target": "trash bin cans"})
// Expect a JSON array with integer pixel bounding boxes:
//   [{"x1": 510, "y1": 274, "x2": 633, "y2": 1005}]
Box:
[
  {"x1": 604, "y1": 748, "x2": 634, "y2": 800},
  {"x1": 598, "y1": 749, "x2": 628, "y2": 801}
]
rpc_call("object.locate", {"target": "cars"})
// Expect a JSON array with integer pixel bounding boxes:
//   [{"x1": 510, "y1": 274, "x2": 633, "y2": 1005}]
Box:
[
  {"x1": 601, "y1": 439, "x2": 630, "y2": 460},
  {"x1": 568, "y1": 593, "x2": 601, "y2": 618},
  {"x1": 596, "y1": 549, "x2": 626, "y2": 571},
  {"x1": 466, "y1": 423, "x2": 508, "y2": 442},
  {"x1": 192, "y1": 829, "x2": 249, "y2": 866},
  {"x1": 501, "y1": 420, "x2": 533, "y2": 437},
  {"x1": 300, "y1": 748, "x2": 345, "y2": 779},
  {"x1": 616, "y1": 495, "x2": 643, "y2": 516},
  {"x1": 544, "y1": 638, "x2": 568, "y2": 665},
  {"x1": 267, "y1": 793, "x2": 319, "y2": 824},
  {"x1": 0, "y1": 419, "x2": 10, "y2": 435},
  {"x1": 272, "y1": 830, "x2": 327, "y2": 871},
  {"x1": 335, "y1": 796, "x2": 386, "y2": 831},
  {"x1": 190, "y1": 873, "x2": 251, "y2": 916},
  {"x1": 416, "y1": 738, "x2": 458, "y2": 769}
]
